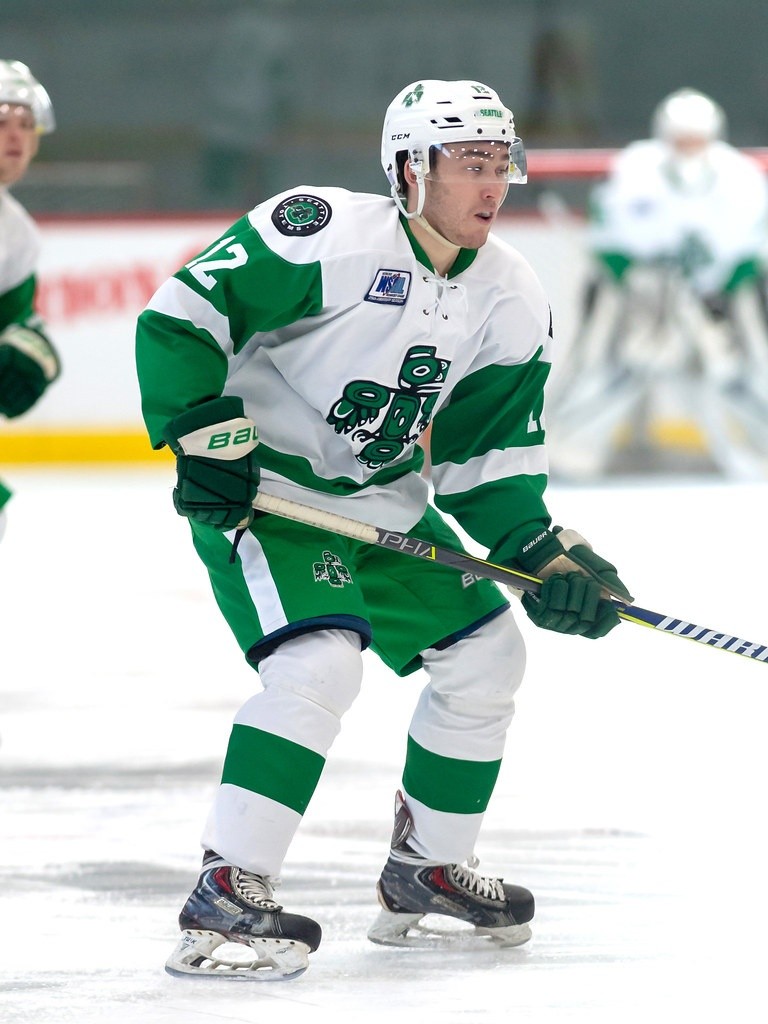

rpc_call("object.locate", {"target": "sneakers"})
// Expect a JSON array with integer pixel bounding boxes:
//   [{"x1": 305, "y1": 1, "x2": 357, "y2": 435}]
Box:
[
  {"x1": 165, "y1": 846, "x2": 321, "y2": 982},
  {"x1": 364, "y1": 791, "x2": 537, "y2": 950}
]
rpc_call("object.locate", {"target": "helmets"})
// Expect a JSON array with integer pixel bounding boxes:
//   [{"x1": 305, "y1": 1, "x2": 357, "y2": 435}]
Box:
[
  {"x1": 660, "y1": 89, "x2": 717, "y2": 137},
  {"x1": 380, "y1": 80, "x2": 516, "y2": 194},
  {"x1": 0, "y1": 58, "x2": 51, "y2": 131}
]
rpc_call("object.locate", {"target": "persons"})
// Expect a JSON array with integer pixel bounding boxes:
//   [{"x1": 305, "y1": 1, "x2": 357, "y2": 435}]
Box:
[
  {"x1": 133, "y1": 77, "x2": 633, "y2": 980},
  {"x1": 542, "y1": 86, "x2": 768, "y2": 479},
  {"x1": 0, "y1": 57, "x2": 61, "y2": 560}
]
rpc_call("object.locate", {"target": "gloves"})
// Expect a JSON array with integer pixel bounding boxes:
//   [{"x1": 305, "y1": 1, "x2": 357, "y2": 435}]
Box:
[
  {"x1": 157, "y1": 392, "x2": 261, "y2": 535},
  {"x1": 1, "y1": 321, "x2": 61, "y2": 416},
  {"x1": 506, "y1": 523, "x2": 635, "y2": 639}
]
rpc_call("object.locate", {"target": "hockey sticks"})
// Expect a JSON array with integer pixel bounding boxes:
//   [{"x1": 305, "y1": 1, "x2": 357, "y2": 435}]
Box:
[{"x1": 249, "y1": 489, "x2": 768, "y2": 669}]
